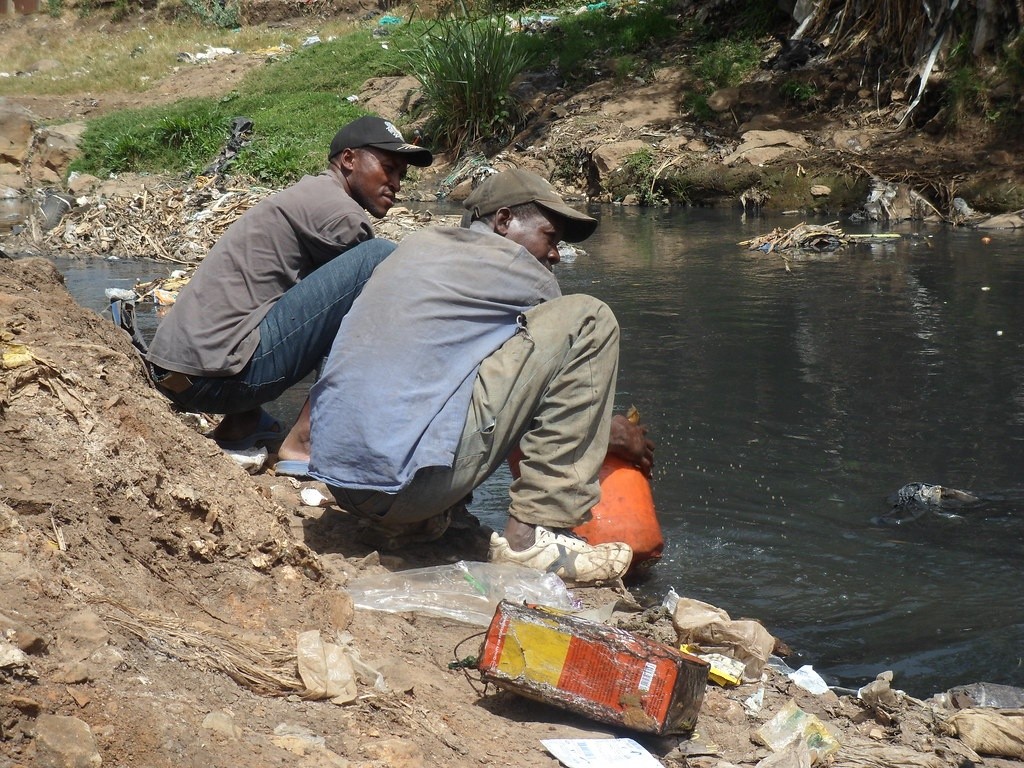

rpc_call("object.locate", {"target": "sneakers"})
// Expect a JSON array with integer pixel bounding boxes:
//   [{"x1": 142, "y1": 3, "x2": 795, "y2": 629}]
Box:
[
  {"x1": 365, "y1": 508, "x2": 451, "y2": 546},
  {"x1": 489, "y1": 524, "x2": 633, "y2": 589}
]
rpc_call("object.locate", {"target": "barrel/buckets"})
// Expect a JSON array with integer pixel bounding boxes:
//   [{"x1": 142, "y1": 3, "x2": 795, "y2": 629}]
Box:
[{"x1": 509, "y1": 444, "x2": 665, "y2": 572}]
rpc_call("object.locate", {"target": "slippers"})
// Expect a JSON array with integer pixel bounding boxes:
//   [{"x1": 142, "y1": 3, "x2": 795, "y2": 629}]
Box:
[
  {"x1": 214, "y1": 408, "x2": 290, "y2": 450},
  {"x1": 275, "y1": 460, "x2": 309, "y2": 476}
]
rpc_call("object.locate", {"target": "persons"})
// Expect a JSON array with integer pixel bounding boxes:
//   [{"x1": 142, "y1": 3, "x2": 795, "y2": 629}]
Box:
[
  {"x1": 309, "y1": 168, "x2": 655, "y2": 585},
  {"x1": 145, "y1": 117, "x2": 433, "y2": 477}
]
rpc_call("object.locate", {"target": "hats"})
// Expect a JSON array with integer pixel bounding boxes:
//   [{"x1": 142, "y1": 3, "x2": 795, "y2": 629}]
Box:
[
  {"x1": 327, "y1": 116, "x2": 433, "y2": 167},
  {"x1": 461, "y1": 169, "x2": 598, "y2": 244}
]
